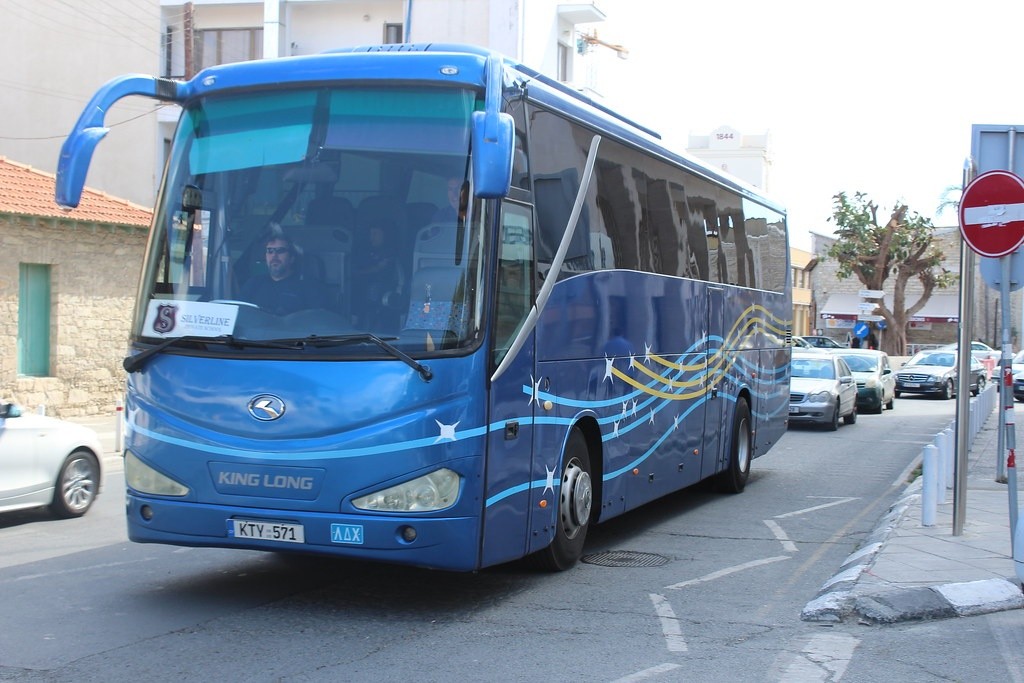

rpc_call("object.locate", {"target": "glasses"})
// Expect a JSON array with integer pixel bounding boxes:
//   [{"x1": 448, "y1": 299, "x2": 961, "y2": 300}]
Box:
[{"x1": 268, "y1": 247, "x2": 289, "y2": 254}]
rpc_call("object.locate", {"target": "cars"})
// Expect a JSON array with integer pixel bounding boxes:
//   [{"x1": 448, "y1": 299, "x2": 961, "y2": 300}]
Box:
[
  {"x1": 788, "y1": 353, "x2": 859, "y2": 431},
  {"x1": 827, "y1": 349, "x2": 896, "y2": 414},
  {"x1": 991, "y1": 350, "x2": 1024, "y2": 392},
  {"x1": 802, "y1": 336, "x2": 846, "y2": 349},
  {"x1": 1012, "y1": 371, "x2": 1024, "y2": 402},
  {"x1": 937, "y1": 341, "x2": 1017, "y2": 367},
  {"x1": 0, "y1": 399, "x2": 106, "y2": 520},
  {"x1": 894, "y1": 350, "x2": 988, "y2": 400},
  {"x1": 792, "y1": 336, "x2": 830, "y2": 370}
]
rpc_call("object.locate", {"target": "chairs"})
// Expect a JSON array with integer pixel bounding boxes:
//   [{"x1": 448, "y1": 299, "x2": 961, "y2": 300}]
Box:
[{"x1": 401, "y1": 266, "x2": 471, "y2": 340}]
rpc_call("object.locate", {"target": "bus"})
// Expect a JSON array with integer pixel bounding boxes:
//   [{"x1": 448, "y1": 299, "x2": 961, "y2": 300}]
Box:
[{"x1": 54, "y1": 42, "x2": 794, "y2": 574}]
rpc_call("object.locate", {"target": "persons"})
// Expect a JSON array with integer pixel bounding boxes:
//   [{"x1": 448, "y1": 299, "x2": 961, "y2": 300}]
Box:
[
  {"x1": 429, "y1": 171, "x2": 469, "y2": 236},
  {"x1": 869, "y1": 334, "x2": 876, "y2": 350},
  {"x1": 850, "y1": 338, "x2": 859, "y2": 348},
  {"x1": 242, "y1": 236, "x2": 317, "y2": 315}
]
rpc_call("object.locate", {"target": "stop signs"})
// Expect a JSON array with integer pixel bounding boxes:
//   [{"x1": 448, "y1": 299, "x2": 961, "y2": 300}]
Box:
[{"x1": 958, "y1": 170, "x2": 1024, "y2": 258}]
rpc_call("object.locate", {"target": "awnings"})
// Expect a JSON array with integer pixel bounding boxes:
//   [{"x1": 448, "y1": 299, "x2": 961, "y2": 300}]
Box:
[{"x1": 820, "y1": 293, "x2": 960, "y2": 323}]
